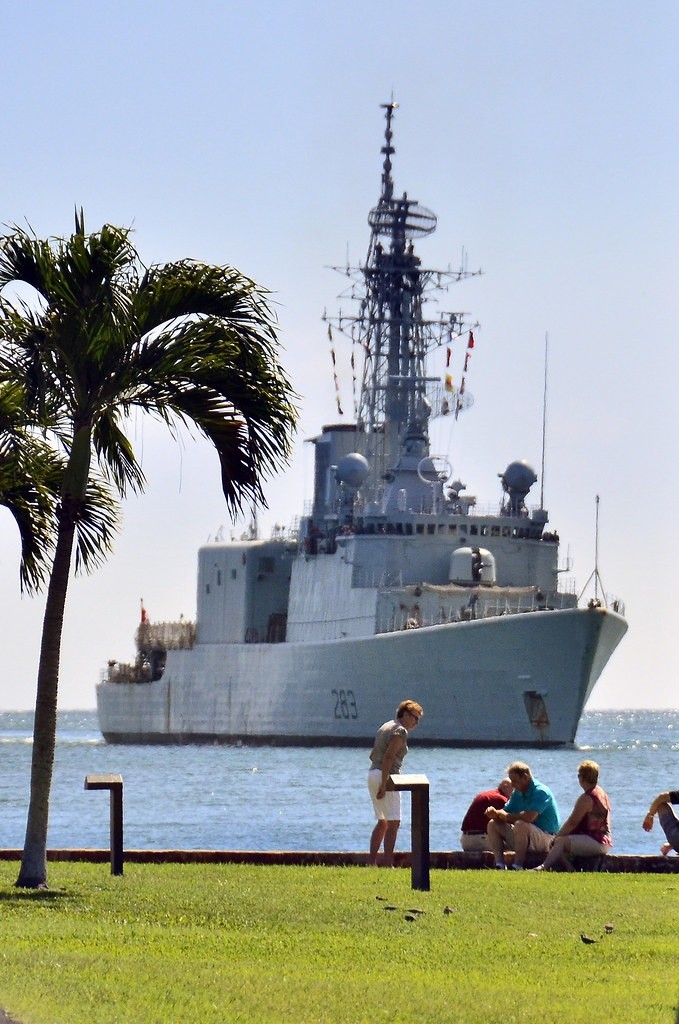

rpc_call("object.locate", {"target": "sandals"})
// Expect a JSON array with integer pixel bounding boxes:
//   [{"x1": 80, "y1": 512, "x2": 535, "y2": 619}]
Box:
[{"x1": 533, "y1": 865, "x2": 544, "y2": 871}]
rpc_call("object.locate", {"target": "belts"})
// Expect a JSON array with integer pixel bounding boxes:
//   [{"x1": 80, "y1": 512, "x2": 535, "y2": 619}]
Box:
[{"x1": 464, "y1": 830, "x2": 486, "y2": 835}]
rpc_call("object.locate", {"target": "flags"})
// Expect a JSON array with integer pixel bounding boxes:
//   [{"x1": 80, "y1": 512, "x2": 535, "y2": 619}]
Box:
[
  {"x1": 454, "y1": 331, "x2": 474, "y2": 421},
  {"x1": 350, "y1": 324, "x2": 358, "y2": 413},
  {"x1": 440, "y1": 331, "x2": 454, "y2": 416},
  {"x1": 328, "y1": 323, "x2": 343, "y2": 416}
]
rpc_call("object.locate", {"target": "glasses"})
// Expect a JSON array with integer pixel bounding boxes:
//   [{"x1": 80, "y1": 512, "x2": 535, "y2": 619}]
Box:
[
  {"x1": 411, "y1": 713, "x2": 420, "y2": 721},
  {"x1": 578, "y1": 775, "x2": 580, "y2": 777}
]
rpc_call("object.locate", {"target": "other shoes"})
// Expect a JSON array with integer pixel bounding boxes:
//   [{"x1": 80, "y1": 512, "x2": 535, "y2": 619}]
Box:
[
  {"x1": 509, "y1": 866, "x2": 515, "y2": 870},
  {"x1": 494, "y1": 865, "x2": 505, "y2": 870}
]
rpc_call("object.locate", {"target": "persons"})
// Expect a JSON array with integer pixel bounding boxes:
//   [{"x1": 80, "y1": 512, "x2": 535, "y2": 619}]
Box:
[
  {"x1": 586, "y1": 598, "x2": 601, "y2": 608},
  {"x1": 367, "y1": 700, "x2": 423, "y2": 868},
  {"x1": 642, "y1": 789, "x2": 679, "y2": 856},
  {"x1": 484, "y1": 762, "x2": 560, "y2": 871},
  {"x1": 530, "y1": 760, "x2": 613, "y2": 872},
  {"x1": 460, "y1": 777, "x2": 516, "y2": 851}
]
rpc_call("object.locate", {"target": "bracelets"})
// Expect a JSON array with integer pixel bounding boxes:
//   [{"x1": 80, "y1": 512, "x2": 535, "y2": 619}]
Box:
[{"x1": 647, "y1": 813, "x2": 654, "y2": 818}]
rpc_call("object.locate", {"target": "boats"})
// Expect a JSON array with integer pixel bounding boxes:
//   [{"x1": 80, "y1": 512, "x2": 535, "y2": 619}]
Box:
[{"x1": 93, "y1": 85, "x2": 631, "y2": 747}]
